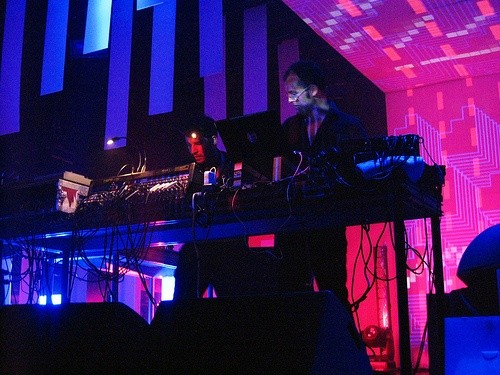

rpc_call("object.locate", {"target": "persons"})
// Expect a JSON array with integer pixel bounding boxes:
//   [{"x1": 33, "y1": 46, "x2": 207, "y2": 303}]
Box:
[
  {"x1": 271, "y1": 60, "x2": 374, "y2": 308},
  {"x1": 171, "y1": 116, "x2": 268, "y2": 299}
]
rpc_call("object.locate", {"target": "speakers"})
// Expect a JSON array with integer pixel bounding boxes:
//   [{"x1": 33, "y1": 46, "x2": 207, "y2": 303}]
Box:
[{"x1": 0, "y1": 292, "x2": 378, "y2": 375}]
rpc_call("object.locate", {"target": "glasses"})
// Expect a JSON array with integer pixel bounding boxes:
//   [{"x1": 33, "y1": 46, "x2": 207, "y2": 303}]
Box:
[{"x1": 285, "y1": 85, "x2": 311, "y2": 100}]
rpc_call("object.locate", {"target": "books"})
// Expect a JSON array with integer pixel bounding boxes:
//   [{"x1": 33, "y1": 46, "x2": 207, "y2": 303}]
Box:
[{"x1": 31, "y1": 170, "x2": 92, "y2": 199}]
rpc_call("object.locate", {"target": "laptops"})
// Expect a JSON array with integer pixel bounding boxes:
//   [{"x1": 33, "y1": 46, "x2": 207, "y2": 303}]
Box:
[{"x1": 216, "y1": 109, "x2": 297, "y2": 165}]
